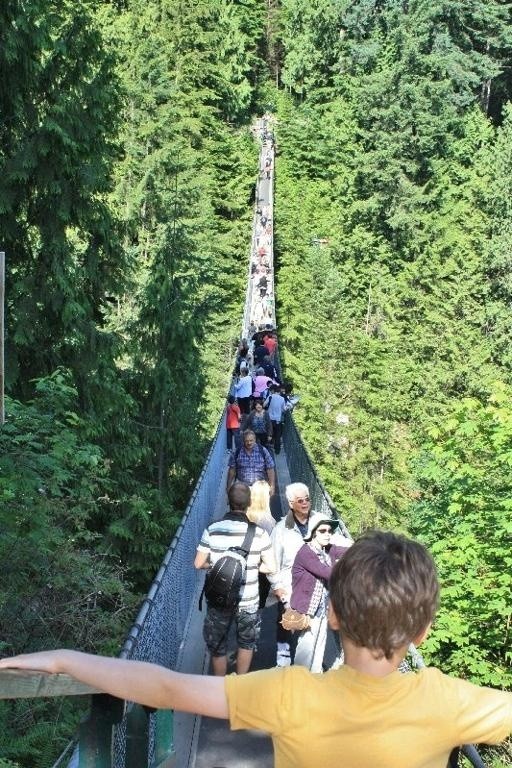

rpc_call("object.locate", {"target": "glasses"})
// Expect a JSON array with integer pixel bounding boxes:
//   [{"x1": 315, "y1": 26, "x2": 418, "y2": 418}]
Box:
[
  {"x1": 297, "y1": 497, "x2": 309, "y2": 503},
  {"x1": 317, "y1": 530, "x2": 334, "y2": 534}
]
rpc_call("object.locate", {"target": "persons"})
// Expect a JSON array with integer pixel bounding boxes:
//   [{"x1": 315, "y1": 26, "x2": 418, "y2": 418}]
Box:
[
  {"x1": 192, "y1": 481, "x2": 279, "y2": 677},
  {"x1": 260, "y1": 481, "x2": 354, "y2": 665},
  {"x1": 291, "y1": 514, "x2": 359, "y2": 675},
  {"x1": 219, "y1": 130, "x2": 298, "y2": 499},
  {"x1": 242, "y1": 477, "x2": 283, "y2": 539},
  {"x1": 0, "y1": 525, "x2": 511, "y2": 768}
]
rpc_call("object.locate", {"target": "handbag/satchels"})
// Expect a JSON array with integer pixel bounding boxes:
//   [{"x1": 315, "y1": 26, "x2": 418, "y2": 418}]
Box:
[
  {"x1": 206, "y1": 523, "x2": 256, "y2": 612},
  {"x1": 281, "y1": 607, "x2": 310, "y2": 630}
]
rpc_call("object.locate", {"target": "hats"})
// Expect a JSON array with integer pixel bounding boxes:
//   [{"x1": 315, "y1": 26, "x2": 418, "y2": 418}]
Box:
[{"x1": 303, "y1": 514, "x2": 339, "y2": 542}]
